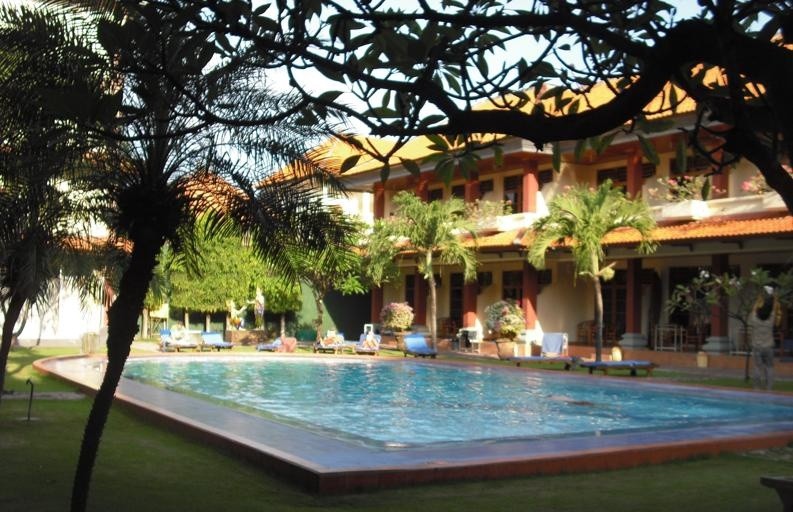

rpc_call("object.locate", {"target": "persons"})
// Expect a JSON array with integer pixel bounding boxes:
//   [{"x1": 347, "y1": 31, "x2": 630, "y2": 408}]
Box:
[
  {"x1": 225, "y1": 299, "x2": 246, "y2": 330},
  {"x1": 169, "y1": 320, "x2": 187, "y2": 343},
  {"x1": 750, "y1": 286, "x2": 780, "y2": 391},
  {"x1": 361, "y1": 331, "x2": 378, "y2": 348},
  {"x1": 245, "y1": 287, "x2": 264, "y2": 330},
  {"x1": 317, "y1": 330, "x2": 342, "y2": 348}
]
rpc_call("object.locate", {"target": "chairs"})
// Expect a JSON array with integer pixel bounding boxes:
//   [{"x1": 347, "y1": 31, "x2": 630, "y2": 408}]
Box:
[
  {"x1": 313, "y1": 333, "x2": 381, "y2": 354},
  {"x1": 159, "y1": 327, "x2": 234, "y2": 352},
  {"x1": 403, "y1": 333, "x2": 438, "y2": 358},
  {"x1": 256, "y1": 337, "x2": 281, "y2": 352}
]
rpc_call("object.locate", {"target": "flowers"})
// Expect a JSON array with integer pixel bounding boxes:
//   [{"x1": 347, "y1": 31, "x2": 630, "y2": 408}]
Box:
[
  {"x1": 741, "y1": 162, "x2": 793, "y2": 195},
  {"x1": 484, "y1": 298, "x2": 528, "y2": 340},
  {"x1": 462, "y1": 197, "x2": 514, "y2": 223},
  {"x1": 379, "y1": 301, "x2": 415, "y2": 330},
  {"x1": 647, "y1": 174, "x2": 727, "y2": 204}
]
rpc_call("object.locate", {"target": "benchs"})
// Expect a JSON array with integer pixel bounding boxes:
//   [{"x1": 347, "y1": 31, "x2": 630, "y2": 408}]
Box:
[{"x1": 511, "y1": 353, "x2": 659, "y2": 376}]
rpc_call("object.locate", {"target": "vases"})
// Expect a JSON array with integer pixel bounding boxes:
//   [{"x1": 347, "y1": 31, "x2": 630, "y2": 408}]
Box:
[
  {"x1": 393, "y1": 331, "x2": 413, "y2": 350},
  {"x1": 495, "y1": 337, "x2": 515, "y2": 361}
]
